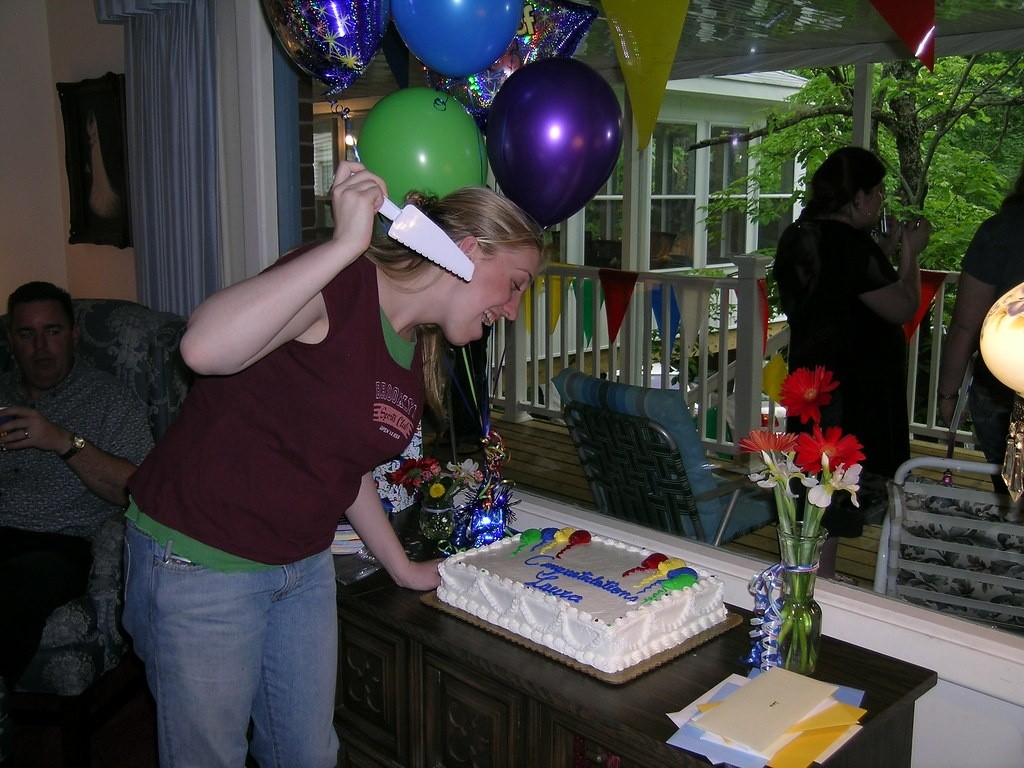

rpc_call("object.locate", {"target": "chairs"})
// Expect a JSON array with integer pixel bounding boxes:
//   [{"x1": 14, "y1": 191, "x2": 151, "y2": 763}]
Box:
[
  {"x1": 872, "y1": 455, "x2": 1024, "y2": 633},
  {"x1": 556, "y1": 369, "x2": 776, "y2": 554}
]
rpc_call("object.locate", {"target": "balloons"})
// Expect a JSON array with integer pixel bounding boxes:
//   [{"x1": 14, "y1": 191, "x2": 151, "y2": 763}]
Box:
[{"x1": 258, "y1": 1, "x2": 626, "y2": 228}]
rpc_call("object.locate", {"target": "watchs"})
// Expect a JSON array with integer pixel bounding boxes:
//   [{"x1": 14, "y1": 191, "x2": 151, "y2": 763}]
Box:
[{"x1": 59, "y1": 433, "x2": 87, "y2": 460}]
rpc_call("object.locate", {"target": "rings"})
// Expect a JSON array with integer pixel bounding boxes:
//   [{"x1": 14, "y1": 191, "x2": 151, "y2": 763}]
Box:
[{"x1": 23, "y1": 429, "x2": 29, "y2": 439}]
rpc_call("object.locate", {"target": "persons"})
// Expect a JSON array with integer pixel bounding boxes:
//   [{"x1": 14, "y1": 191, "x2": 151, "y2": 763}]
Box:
[
  {"x1": 120, "y1": 161, "x2": 545, "y2": 768},
  {"x1": 0, "y1": 281, "x2": 154, "y2": 686},
  {"x1": 772, "y1": 147, "x2": 933, "y2": 586},
  {"x1": 939, "y1": 162, "x2": 1024, "y2": 495}
]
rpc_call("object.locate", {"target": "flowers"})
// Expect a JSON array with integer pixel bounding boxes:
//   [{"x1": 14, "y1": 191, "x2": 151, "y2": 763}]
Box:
[{"x1": 739, "y1": 351, "x2": 867, "y2": 674}]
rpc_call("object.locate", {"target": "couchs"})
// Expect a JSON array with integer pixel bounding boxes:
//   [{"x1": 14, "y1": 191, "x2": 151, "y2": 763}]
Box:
[{"x1": 1, "y1": 297, "x2": 207, "y2": 741}]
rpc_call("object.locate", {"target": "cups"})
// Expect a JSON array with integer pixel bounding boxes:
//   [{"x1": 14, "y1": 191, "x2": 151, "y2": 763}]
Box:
[
  {"x1": 877, "y1": 202, "x2": 892, "y2": 236},
  {"x1": 0, "y1": 401, "x2": 17, "y2": 450}
]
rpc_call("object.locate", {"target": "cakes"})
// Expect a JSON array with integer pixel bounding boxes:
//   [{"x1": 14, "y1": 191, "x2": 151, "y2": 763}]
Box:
[{"x1": 436, "y1": 528, "x2": 729, "y2": 675}]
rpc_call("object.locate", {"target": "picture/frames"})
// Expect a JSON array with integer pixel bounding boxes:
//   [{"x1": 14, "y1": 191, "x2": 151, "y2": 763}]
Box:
[{"x1": 57, "y1": 71, "x2": 133, "y2": 249}]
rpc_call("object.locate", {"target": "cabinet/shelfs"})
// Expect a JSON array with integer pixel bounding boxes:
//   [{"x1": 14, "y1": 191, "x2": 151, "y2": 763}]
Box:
[{"x1": 333, "y1": 551, "x2": 938, "y2": 768}]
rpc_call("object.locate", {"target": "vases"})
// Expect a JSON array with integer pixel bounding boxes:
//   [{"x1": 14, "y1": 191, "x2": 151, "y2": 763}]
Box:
[{"x1": 775, "y1": 524, "x2": 830, "y2": 670}]
